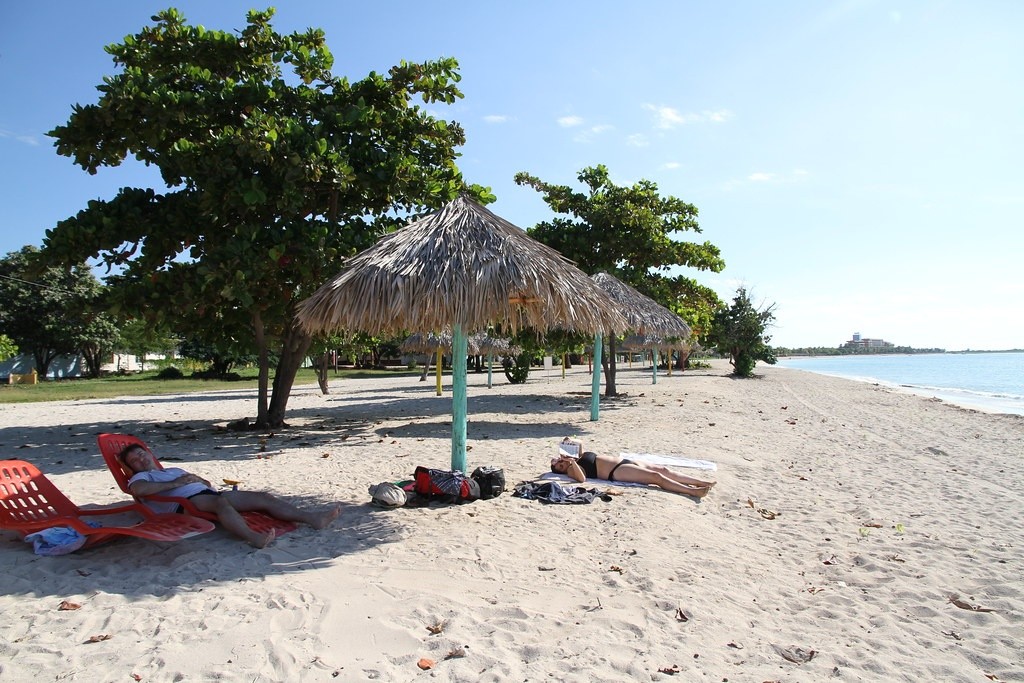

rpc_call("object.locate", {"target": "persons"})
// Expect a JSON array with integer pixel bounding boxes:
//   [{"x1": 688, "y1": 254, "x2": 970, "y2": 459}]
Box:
[
  {"x1": 551, "y1": 437, "x2": 718, "y2": 498},
  {"x1": 118, "y1": 442, "x2": 341, "y2": 550}
]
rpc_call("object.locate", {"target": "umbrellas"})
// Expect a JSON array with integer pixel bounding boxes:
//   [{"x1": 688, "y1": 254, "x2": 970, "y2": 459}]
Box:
[
  {"x1": 291, "y1": 193, "x2": 626, "y2": 496},
  {"x1": 539, "y1": 269, "x2": 690, "y2": 420}
]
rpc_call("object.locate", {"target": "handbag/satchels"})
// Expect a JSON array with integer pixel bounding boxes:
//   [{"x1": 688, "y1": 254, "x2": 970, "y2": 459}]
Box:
[
  {"x1": 414, "y1": 466, "x2": 481, "y2": 502},
  {"x1": 472, "y1": 467, "x2": 505, "y2": 497}
]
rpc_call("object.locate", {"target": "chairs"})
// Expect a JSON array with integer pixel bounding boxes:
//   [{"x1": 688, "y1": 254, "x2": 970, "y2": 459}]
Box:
[
  {"x1": 0, "y1": 458, "x2": 216, "y2": 541},
  {"x1": 97, "y1": 433, "x2": 300, "y2": 538}
]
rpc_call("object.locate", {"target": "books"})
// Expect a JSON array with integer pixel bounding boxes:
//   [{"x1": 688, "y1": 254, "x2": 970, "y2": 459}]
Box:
[{"x1": 559, "y1": 440, "x2": 581, "y2": 458}]
